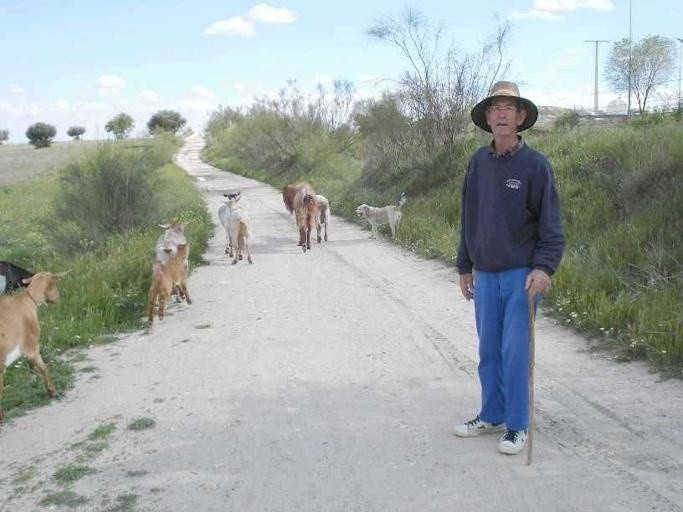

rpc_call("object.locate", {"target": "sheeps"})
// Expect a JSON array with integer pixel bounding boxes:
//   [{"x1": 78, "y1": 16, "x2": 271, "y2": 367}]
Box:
[
  {"x1": 218, "y1": 191, "x2": 241, "y2": 254},
  {"x1": 220, "y1": 195, "x2": 254, "y2": 266},
  {"x1": 0, "y1": 267, "x2": 74, "y2": 408},
  {"x1": 150, "y1": 219, "x2": 197, "y2": 303},
  {"x1": 0, "y1": 259, "x2": 34, "y2": 295},
  {"x1": 146, "y1": 240, "x2": 193, "y2": 326},
  {"x1": 279, "y1": 181, "x2": 317, "y2": 253},
  {"x1": 315, "y1": 194, "x2": 331, "y2": 243}
]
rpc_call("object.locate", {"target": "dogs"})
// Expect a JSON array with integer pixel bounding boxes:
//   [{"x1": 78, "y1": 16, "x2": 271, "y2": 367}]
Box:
[{"x1": 354, "y1": 190, "x2": 408, "y2": 241}]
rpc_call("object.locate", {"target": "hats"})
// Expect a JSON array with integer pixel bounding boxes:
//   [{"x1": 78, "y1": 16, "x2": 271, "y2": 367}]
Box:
[{"x1": 470, "y1": 80, "x2": 539, "y2": 134}]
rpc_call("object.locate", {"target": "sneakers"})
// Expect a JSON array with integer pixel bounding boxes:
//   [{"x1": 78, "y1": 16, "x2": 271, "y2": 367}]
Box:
[
  {"x1": 497, "y1": 423, "x2": 530, "y2": 456},
  {"x1": 453, "y1": 414, "x2": 508, "y2": 439}
]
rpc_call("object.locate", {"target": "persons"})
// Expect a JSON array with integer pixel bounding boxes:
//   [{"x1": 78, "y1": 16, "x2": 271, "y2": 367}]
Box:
[{"x1": 452, "y1": 81, "x2": 566, "y2": 455}]
